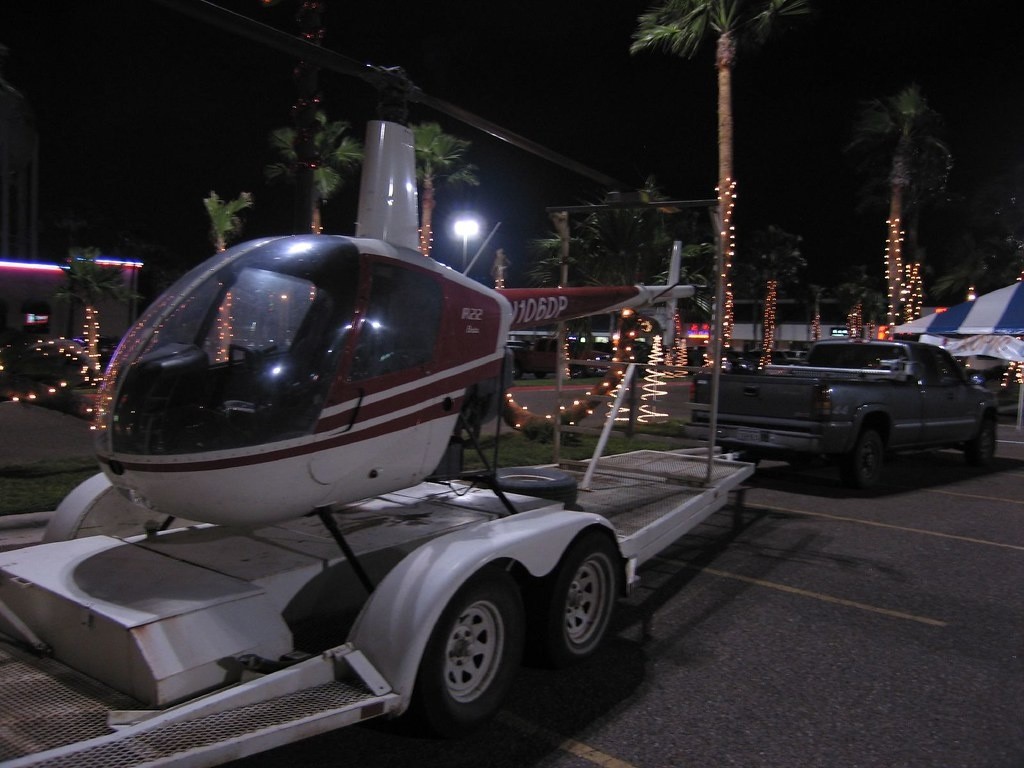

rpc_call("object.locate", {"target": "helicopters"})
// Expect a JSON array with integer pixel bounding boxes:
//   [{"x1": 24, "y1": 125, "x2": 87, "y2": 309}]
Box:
[{"x1": 0, "y1": 1, "x2": 712, "y2": 672}]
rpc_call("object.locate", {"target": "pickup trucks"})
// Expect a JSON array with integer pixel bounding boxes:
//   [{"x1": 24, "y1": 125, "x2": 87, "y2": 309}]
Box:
[{"x1": 682, "y1": 338, "x2": 1002, "y2": 495}]
